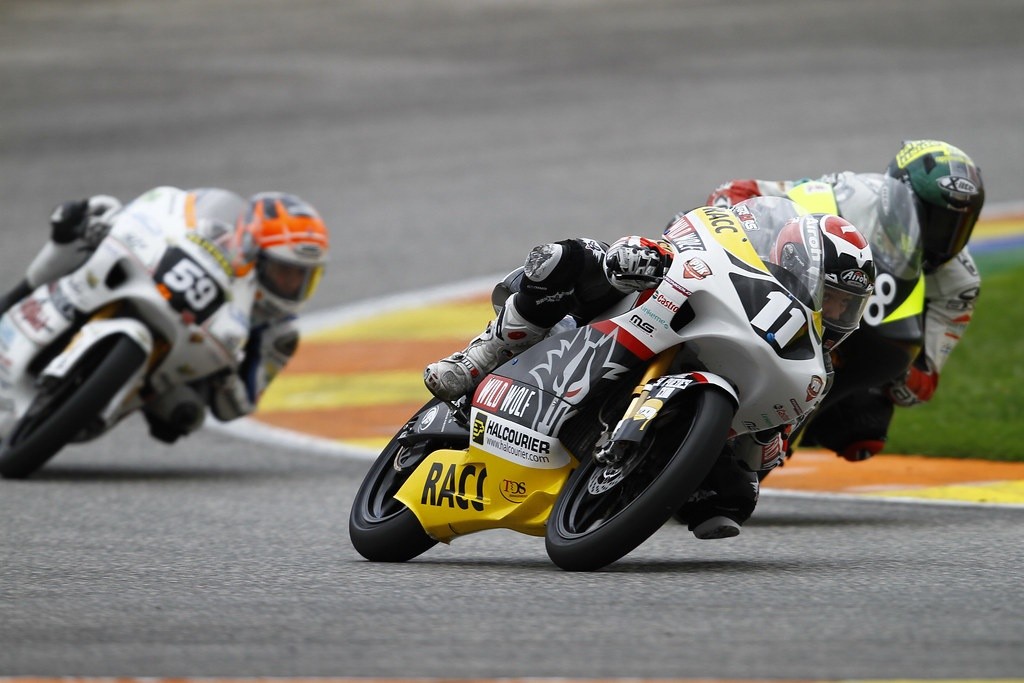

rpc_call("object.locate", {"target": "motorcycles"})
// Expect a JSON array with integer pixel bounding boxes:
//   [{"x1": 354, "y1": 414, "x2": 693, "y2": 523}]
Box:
[
  {"x1": 350, "y1": 194, "x2": 829, "y2": 574},
  {"x1": 1, "y1": 186, "x2": 261, "y2": 478},
  {"x1": 772, "y1": 172, "x2": 922, "y2": 458}
]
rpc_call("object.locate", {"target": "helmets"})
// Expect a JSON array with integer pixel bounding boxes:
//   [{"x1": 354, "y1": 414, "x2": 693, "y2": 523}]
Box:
[
  {"x1": 879, "y1": 139, "x2": 985, "y2": 276},
  {"x1": 768, "y1": 213, "x2": 878, "y2": 357},
  {"x1": 231, "y1": 191, "x2": 333, "y2": 321}
]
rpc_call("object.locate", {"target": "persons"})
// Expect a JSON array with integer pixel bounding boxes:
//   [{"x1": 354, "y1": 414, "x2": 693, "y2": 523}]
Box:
[
  {"x1": 425, "y1": 214, "x2": 876, "y2": 540},
  {"x1": 707, "y1": 139, "x2": 983, "y2": 463},
  {"x1": 0, "y1": 190, "x2": 330, "y2": 446}
]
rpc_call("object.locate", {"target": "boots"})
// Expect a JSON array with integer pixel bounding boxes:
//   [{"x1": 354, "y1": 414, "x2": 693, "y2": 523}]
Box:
[{"x1": 423, "y1": 291, "x2": 552, "y2": 404}]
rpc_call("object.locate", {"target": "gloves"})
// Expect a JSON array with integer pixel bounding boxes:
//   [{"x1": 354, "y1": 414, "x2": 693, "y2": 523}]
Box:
[
  {"x1": 733, "y1": 429, "x2": 784, "y2": 472},
  {"x1": 602, "y1": 235, "x2": 671, "y2": 295},
  {"x1": 884, "y1": 358, "x2": 938, "y2": 409}
]
